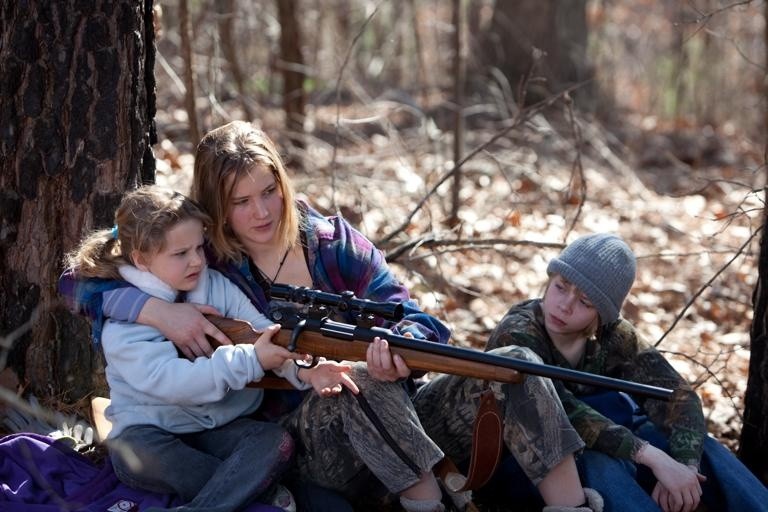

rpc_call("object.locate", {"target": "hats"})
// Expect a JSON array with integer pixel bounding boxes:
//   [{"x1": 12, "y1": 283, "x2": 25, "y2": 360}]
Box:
[{"x1": 547, "y1": 235, "x2": 636, "y2": 326}]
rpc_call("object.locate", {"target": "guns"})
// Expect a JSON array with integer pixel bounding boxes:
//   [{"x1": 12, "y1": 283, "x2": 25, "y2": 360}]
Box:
[{"x1": 174, "y1": 284, "x2": 675, "y2": 401}]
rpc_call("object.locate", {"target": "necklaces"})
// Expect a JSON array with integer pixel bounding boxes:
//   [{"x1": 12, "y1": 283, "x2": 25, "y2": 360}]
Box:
[{"x1": 234, "y1": 240, "x2": 296, "y2": 284}]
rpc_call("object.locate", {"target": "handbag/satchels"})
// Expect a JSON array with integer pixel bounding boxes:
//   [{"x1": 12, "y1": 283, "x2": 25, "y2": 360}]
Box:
[{"x1": 577, "y1": 391, "x2": 648, "y2": 432}]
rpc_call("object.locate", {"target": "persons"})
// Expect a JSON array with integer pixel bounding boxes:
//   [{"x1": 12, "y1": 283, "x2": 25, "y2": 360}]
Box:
[
  {"x1": 55, "y1": 119, "x2": 605, "y2": 512},
  {"x1": 484, "y1": 232, "x2": 768, "y2": 512},
  {"x1": 63, "y1": 182, "x2": 360, "y2": 512}
]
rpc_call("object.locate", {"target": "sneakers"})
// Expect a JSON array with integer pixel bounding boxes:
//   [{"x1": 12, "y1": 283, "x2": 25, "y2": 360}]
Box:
[{"x1": 259, "y1": 479, "x2": 297, "y2": 512}]
